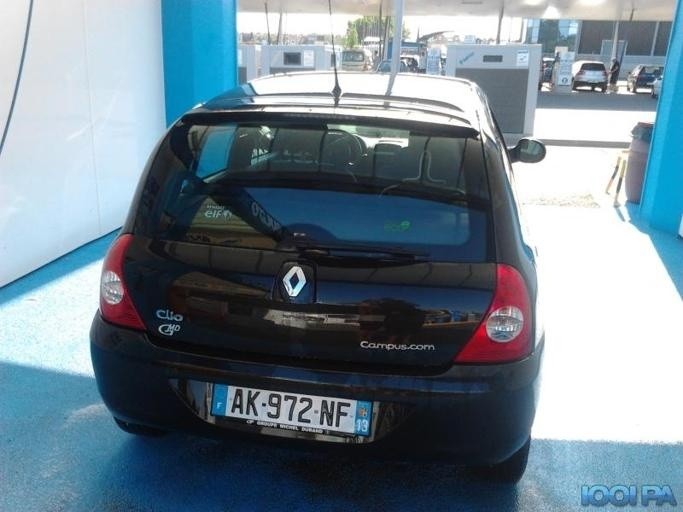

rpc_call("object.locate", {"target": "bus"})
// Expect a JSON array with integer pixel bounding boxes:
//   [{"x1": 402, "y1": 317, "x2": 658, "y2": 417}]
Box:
[{"x1": 342, "y1": 48, "x2": 373, "y2": 70}]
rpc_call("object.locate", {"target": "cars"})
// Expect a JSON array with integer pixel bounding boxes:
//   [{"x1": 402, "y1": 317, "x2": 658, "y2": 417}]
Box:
[
  {"x1": 628, "y1": 63, "x2": 665, "y2": 97},
  {"x1": 571, "y1": 60, "x2": 609, "y2": 92},
  {"x1": 90, "y1": 72, "x2": 547, "y2": 484}
]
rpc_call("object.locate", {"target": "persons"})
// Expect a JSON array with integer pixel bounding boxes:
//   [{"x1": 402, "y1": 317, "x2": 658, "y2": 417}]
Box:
[
  {"x1": 609, "y1": 55, "x2": 620, "y2": 92},
  {"x1": 371, "y1": 50, "x2": 380, "y2": 69}
]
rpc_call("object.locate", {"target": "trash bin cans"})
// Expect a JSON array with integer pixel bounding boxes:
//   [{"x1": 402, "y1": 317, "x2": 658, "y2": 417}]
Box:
[{"x1": 625, "y1": 122, "x2": 654, "y2": 204}]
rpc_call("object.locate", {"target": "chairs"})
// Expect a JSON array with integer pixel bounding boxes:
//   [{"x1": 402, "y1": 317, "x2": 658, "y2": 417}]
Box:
[{"x1": 213, "y1": 123, "x2": 466, "y2": 245}]
